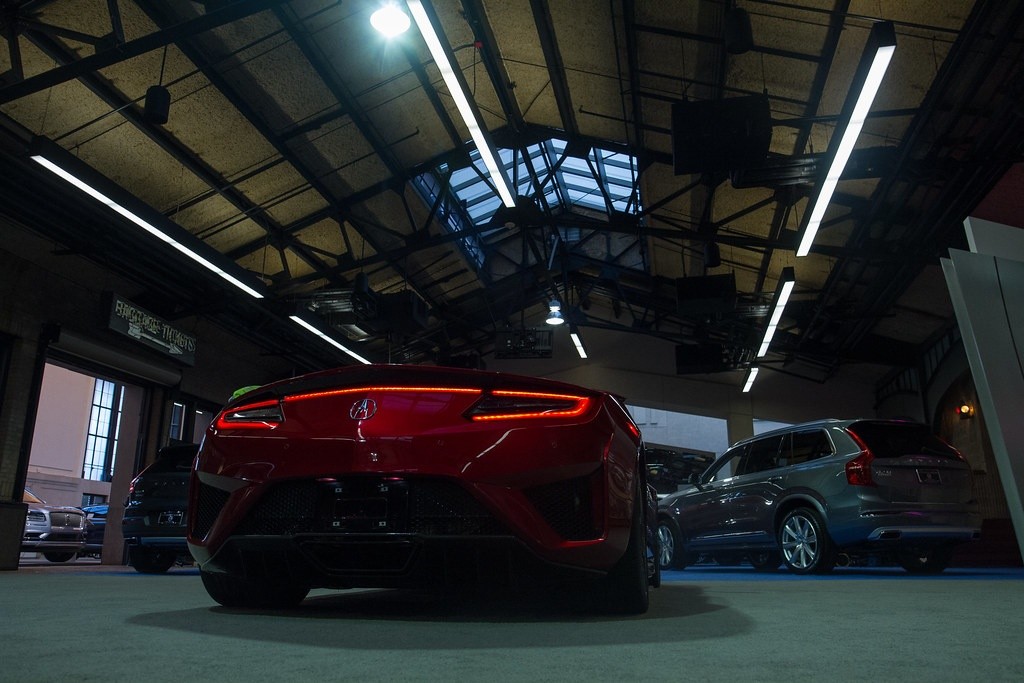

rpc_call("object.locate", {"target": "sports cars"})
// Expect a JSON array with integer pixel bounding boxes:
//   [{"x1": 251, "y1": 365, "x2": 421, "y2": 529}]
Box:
[{"x1": 186, "y1": 361, "x2": 663, "y2": 617}]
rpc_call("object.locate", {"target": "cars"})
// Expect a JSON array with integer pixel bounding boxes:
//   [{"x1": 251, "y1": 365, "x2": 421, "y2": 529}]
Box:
[{"x1": 78, "y1": 503, "x2": 109, "y2": 555}]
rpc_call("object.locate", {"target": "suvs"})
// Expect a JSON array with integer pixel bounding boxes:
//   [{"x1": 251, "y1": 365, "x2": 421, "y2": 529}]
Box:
[
  {"x1": 22, "y1": 486, "x2": 87, "y2": 563},
  {"x1": 121, "y1": 445, "x2": 199, "y2": 575},
  {"x1": 659, "y1": 417, "x2": 987, "y2": 575}
]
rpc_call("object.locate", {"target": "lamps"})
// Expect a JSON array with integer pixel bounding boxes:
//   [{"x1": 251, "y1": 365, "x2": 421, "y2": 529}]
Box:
[
  {"x1": 288, "y1": 309, "x2": 376, "y2": 365},
  {"x1": 352, "y1": 238, "x2": 370, "y2": 297},
  {"x1": 30, "y1": 65, "x2": 268, "y2": 299},
  {"x1": 756, "y1": 251, "x2": 796, "y2": 358},
  {"x1": 792, "y1": 0, "x2": 897, "y2": 257},
  {"x1": 142, "y1": 46, "x2": 172, "y2": 125},
  {"x1": 741, "y1": 365, "x2": 760, "y2": 393},
  {"x1": 723, "y1": 0, "x2": 753, "y2": 54},
  {"x1": 569, "y1": 327, "x2": 589, "y2": 359},
  {"x1": 704, "y1": 192, "x2": 721, "y2": 268}
]
